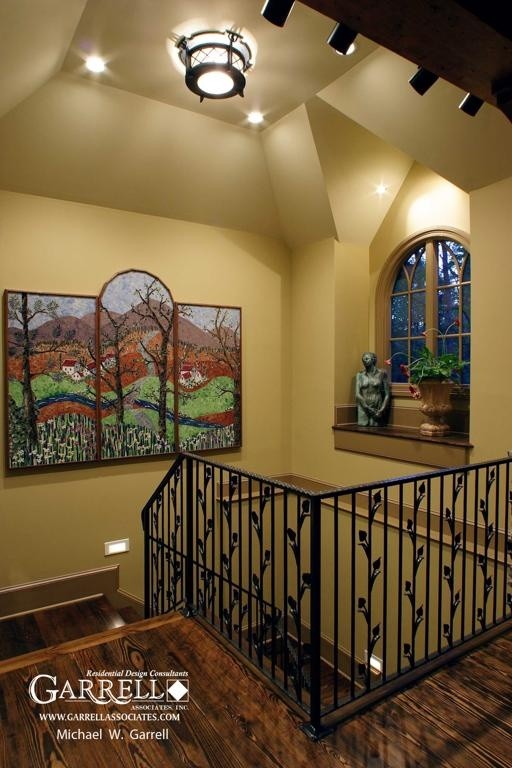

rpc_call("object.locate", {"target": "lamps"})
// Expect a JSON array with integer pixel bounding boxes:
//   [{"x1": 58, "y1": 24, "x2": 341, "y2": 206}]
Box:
[
  {"x1": 261, "y1": 0, "x2": 358, "y2": 56},
  {"x1": 172, "y1": 27, "x2": 255, "y2": 105},
  {"x1": 409, "y1": 63, "x2": 487, "y2": 116}
]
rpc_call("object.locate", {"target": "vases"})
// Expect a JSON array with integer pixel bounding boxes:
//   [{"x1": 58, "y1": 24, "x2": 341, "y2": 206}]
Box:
[{"x1": 415, "y1": 382, "x2": 455, "y2": 437}]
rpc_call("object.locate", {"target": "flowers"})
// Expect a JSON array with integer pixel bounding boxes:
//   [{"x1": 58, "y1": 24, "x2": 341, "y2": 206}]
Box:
[{"x1": 384, "y1": 320, "x2": 469, "y2": 400}]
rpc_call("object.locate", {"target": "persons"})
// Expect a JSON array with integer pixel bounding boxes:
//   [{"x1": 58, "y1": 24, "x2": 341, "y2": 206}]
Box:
[{"x1": 351, "y1": 350, "x2": 393, "y2": 429}]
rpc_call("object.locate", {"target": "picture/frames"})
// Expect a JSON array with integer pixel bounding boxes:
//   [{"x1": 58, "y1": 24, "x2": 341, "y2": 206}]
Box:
[{"x1": 2, "y1": 268, "x2": 243, "y2": 476}]
